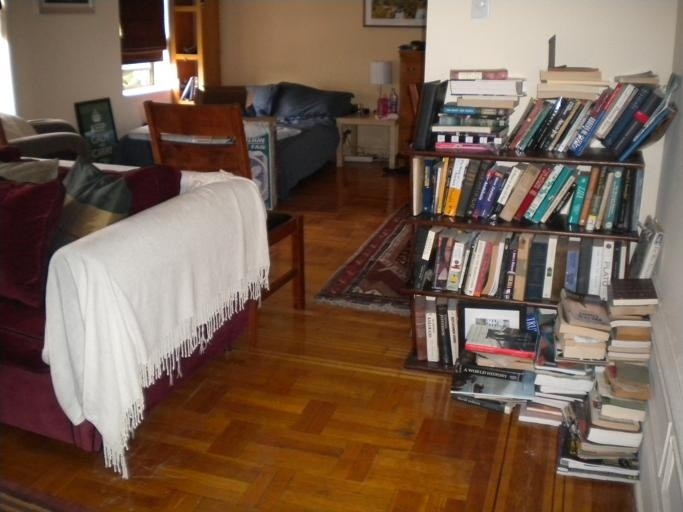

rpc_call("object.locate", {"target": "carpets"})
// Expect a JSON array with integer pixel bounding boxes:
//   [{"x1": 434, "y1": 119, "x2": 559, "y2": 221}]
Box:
[{"x1": 313, "y1": 206, "x2": 415, "y2": 316}]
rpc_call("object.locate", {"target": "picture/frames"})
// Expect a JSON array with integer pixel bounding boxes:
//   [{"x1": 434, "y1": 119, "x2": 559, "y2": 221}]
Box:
[
  {"x1": 74, "y1": 97, "x2": 120, "y2": 162},
  {"x1": 33, "y1": 0, "x2": 95, "y2": 15},
  {"x1": 362, "y1": 0, "x2": 427, "y2": 28}
]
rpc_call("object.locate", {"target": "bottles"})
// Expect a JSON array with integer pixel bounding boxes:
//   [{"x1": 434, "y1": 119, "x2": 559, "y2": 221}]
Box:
[{"x1": 390, "y1": 88, "x2": 397, "y2": 113}]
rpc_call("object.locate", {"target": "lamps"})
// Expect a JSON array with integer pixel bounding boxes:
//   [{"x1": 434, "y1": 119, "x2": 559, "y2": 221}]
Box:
[{"x1": 369, "y1": 60, "x2": 394, "y2": 99}]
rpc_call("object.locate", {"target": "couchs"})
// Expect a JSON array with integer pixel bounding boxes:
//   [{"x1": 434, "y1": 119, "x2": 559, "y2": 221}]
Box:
[{"x1": 1, "y1": 156, "x2": 272, "y2": 385}]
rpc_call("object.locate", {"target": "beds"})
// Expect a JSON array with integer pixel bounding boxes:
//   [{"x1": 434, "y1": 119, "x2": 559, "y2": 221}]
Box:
[{"x1": 119, "y1": 114, "x2": 340, "y2": 208}]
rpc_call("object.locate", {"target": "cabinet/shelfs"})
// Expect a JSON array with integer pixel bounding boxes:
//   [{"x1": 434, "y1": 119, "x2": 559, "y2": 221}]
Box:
[
  {"x1": 167, "y1": 0, "x2": 220, "y2": 104},
  {"x1": 398, "y1": 50, "x2": 425, "y2": 156},
  {"x1": 405, "y1": 150, "x2": 643, "y2": 375}
]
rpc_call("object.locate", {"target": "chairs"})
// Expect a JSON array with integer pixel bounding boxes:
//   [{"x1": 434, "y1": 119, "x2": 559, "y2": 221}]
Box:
[
  {"x1": 145, "y1": 101, "x2": 307, "y2": 347},
  {"x1": 0, "y1": 114, "x2": 91, "y2": 163}
]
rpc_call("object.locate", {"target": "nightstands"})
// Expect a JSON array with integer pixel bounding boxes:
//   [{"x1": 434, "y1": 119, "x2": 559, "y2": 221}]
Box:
[{"x1": 337, "y1": 114, "x2": 400, "y2": 169}]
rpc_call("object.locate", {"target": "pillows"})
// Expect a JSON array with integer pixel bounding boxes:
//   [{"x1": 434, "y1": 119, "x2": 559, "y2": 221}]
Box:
[
  {"x1": 0, "y1": 178, "x2": 64, "y2": 309},
  {"x1": 203, "y1": 84, "x2": 277, "y2": 116},
  {"x1": 0, "y1": 158, "x2": 59, "y2": 186},
  {"x1": 272, "y1": 81, "x2": 355, "y2": 126},
  {"x1": 1, "y1": 114, "x2": 34, "y2": 140},
  {"x1": 106, "y1": 164, "x2": 183, "y2": 216},
  {"x1": 43, "y1": 156, "x2": 134, "y2": 278}
]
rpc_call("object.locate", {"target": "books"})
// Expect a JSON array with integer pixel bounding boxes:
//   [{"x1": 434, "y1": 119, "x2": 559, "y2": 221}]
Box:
[
  {"x1": 412, "y1": 217, "x2": 663, "y2": 303},
  {"x1": 516, "y1": 287, "x2": 611, "y2": 427},
  {"x1": 413, "y1": 155, "x2": 644, "y2": 232},
  {"x1": 450, "y1": 325, "x2": 543, "y2": 416},
  {"x1": 604, "y1": 278, "x2": 660, "y2": 365},
  {"x1": 413, "y1": 292, "x2": 537, "y2": 367},
  {"x1": 555, "y1": 361, "x2": 650, "y2": 483},
  {"x1": 411, "y1": 71, "x2": 681, "y2": 164}
]
